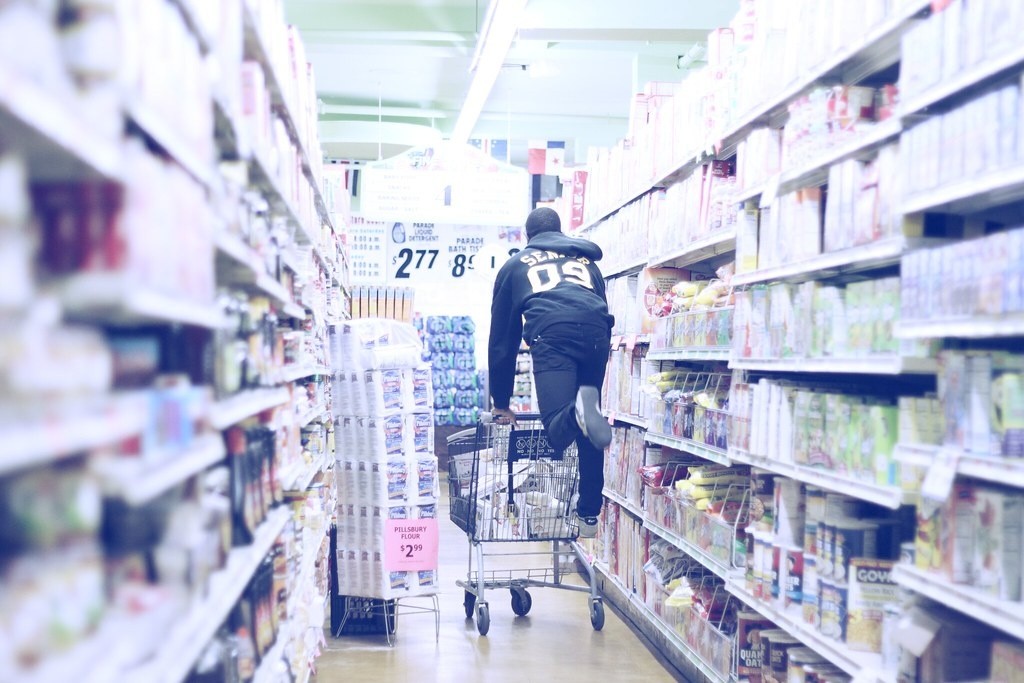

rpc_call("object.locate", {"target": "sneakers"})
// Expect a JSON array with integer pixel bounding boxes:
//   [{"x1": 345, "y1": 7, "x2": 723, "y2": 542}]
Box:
[
  {"x1": 570, "y1": 494, "x2": 598, "y2": 536},
  {"x1": 575, "y1": 386, "x2": 612, "y2": 449}
]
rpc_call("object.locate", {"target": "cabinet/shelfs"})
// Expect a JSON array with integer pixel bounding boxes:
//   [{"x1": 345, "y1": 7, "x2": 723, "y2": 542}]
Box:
[
  {"x1": 567, "y1": 0, "x2": 1023, "y2": 681},
  {"x1": 0, "y1": 0, "x2": 336, "y2": 683}
]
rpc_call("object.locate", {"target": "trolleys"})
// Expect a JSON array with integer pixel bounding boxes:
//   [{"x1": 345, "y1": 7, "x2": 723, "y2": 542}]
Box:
[{"x1": 446, "y1": 408, "x2": 610, "y2": 635}]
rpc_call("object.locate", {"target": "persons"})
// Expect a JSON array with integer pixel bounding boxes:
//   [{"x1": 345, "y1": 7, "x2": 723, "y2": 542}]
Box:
[{"x1": 487, "y1": 207, "x2": 615, "y2": 539}]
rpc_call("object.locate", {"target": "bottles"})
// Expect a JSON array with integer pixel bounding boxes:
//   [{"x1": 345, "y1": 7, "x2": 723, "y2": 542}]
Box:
[
  {"x1": 187, "y1": 407, "x2": 292, "y2": 683},
  {"x1": 731, "y1": 281, "x2": 819, "y2": 357}
]
